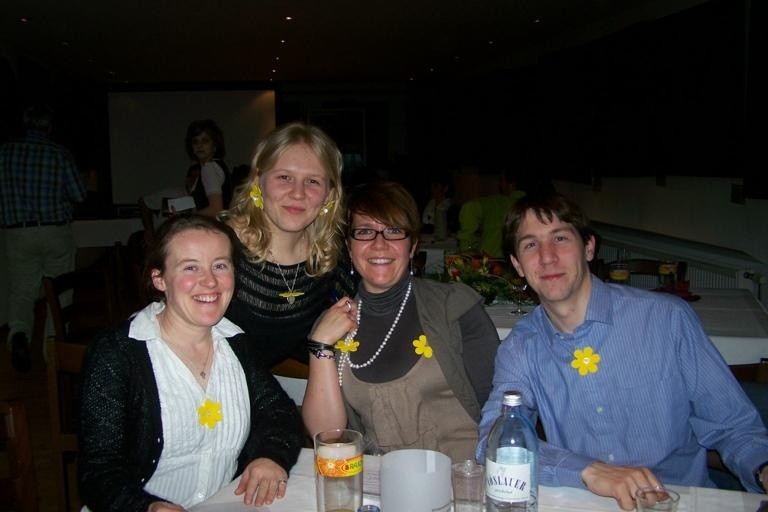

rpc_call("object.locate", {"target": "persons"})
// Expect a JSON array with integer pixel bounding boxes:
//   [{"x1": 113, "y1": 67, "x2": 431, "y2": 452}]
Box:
[
  {"x1": 475, "y1": 191, "x2": 768, "y2": 512},
  {"x1": 0, "y1": 104, "x2": 86, "y2": 377},
  {"x1": 168, "y1": 118, "x2": 231, "y2": 221},
  {"x1": 74, "y1": 210, "x2": 304, "y2": 511},
  {"x1": 300, "y1": 179, "x2": 500, "y2": 466},
  {"x1": 215, "y1": 121, "x2": 360, "y2": 366}
]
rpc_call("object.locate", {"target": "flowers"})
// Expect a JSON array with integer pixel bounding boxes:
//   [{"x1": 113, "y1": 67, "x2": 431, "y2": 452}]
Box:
[
  {"x1": 424, "y1": 251, "x2": 534, "y2": 312},
  {"x1": 566, "y1": 343, "x2": 603, "y2": 377},
  {"x1": 319, "y1": 198, "x2": 337, "y2": 218},
  {"x1": 196, "y1": 397, "x2": 223, "y2": 430},
  {"x1": 409, "y1": 333, "x2": 437, "y2": 361},
  {"x1": 277, "y1": 287, "x2": 307, "y2": 305},
  {"x1": 331, "y1": 341, "x2": 361, "y2": 354},
  {"x1": 247, "y1": 184, "x2": 263, "y2": 211}
]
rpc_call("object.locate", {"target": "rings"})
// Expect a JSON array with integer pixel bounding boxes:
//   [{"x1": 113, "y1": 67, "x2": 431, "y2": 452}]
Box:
[
  {"x1": 279, "y1": 480, "x2": 287, "y2": 485},
  {"x1": 346, "y1": 300, "x2": 352, "y2": 311}
]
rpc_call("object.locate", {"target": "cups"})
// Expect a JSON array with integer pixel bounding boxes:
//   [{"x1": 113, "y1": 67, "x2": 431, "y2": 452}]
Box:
[
  {"x1": 607, "y1": 262, "x2": 630, "y2": 285},
  {"x1": 658, "y1": 259, "x2": 678, "y2": 286},
  {"x1": 449, "y1": 458, "x2": 485, "y2": 511},
  {"x1": 312, "y1": 427, "x2": 365, "y2": 512},
  {"x1": 380, "y1": 446, "x2": 452, "y2": 511},
  {"x1": 634, "y1": 486, "x2": 682, "y2": 511}
]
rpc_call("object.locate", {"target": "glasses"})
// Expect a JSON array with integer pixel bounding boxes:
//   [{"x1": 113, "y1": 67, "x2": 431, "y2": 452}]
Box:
[{"x1": 345, "y1": 225, "x2": 419, "y2": 245}]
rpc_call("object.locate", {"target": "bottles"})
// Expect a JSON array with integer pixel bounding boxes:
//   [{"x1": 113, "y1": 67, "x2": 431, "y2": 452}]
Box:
[{"x1": 483, "y1": 389, "x2": 539, "y2": 512}]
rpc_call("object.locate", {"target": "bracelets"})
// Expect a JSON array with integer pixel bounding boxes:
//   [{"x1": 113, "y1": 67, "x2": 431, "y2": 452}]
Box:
[{"x1": 307, "y1": 339, "x2": 335, "y2": 360}]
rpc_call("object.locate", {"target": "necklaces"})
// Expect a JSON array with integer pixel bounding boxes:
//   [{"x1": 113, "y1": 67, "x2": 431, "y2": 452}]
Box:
[
  {"x1": 268, "y1": 237, "x2": 303, "y2": 305},
  {"x1": 337, "y1": 280, "x2": 412, "y2": 387}
]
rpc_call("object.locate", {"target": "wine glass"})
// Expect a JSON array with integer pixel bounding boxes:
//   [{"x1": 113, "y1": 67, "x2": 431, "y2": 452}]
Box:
[{"x1": 508, "y1": 274, "x2": 529, "y2": 316}]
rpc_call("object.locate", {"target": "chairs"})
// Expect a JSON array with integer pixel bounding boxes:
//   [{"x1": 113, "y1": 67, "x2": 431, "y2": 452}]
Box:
[
  {"x1": 40, "y1": 198, "x2": 152, "y2": 512},
  {"x1": 589, "y1": 252, "x2": 690, "y2": 291}
]
